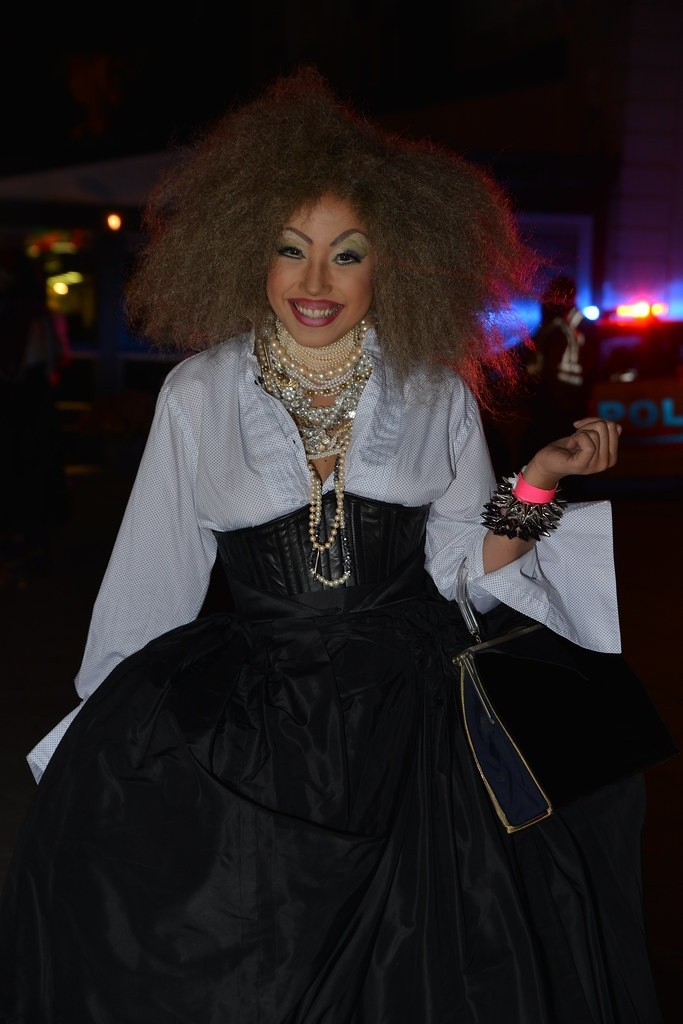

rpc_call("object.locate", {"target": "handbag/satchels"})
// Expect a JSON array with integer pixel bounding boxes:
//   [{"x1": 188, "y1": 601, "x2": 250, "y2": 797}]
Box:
[{"x1": 448, "y1": 556, "x2": 679, "y2": 835}]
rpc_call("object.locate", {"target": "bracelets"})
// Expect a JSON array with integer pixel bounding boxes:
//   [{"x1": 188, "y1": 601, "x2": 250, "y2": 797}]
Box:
[
  {"x1": 512, "y1": 473, "x2": 558, "y2": 504},
  {"x1": 482, "y1": 477, "x2": 566, "y2": 542}
]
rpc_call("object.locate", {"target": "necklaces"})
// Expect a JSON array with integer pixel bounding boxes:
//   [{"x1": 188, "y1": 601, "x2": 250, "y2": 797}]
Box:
[{"x1": 255, "y1": 308, "x2": 376, "y2": 587}]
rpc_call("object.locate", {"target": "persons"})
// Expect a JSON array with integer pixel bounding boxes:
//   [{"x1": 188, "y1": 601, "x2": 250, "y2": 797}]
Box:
[
  {"x1": 18, "y1": 67, "x2": 649, "y2": 1024},
  {"x1": 523, "y1": 270, "x2": 600, "y2": 452}
]
